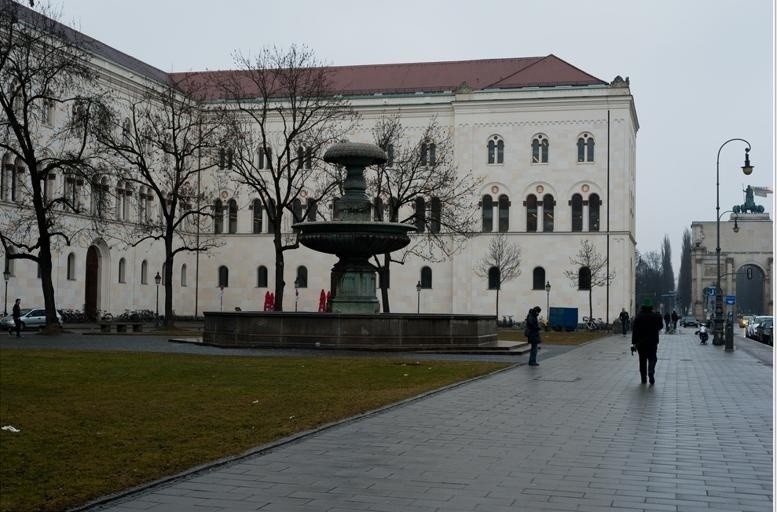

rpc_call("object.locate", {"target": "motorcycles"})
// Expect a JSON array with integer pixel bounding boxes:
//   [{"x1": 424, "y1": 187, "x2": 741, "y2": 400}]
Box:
[{"x1": 700, "y1": 331, "x2": 709, "y2": 345}]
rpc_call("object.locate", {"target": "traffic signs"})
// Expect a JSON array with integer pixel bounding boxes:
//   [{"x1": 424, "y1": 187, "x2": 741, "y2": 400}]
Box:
[
  {"x1": 725, "y1": 295, "x2": 736, "y2": 306},
  {"x1": 708, "y1": 287, "x2": 724, "y2": 297}
]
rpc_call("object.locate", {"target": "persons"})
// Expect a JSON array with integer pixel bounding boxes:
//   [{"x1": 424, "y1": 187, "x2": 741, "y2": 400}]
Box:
[
  {"x1": 618, "y1": 307, "x2": 629, "y2": 337},
  {"x1": 523, "y1": 306, "x2": 542, "y2": 366},
  {"x1": 629, "y1": 305, "x2": 662, "y2": 385},
  {"x1": 647, "y1": 305, "x2": 680, "y2": 332},
  {"x1": 7, "y1": 298, "x2": 23, "y2": 338}
]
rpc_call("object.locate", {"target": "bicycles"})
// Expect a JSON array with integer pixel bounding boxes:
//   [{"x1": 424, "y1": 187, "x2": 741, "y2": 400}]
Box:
[
  {"x1": 58, "y1": 307, "x2": 162, "y2": 322},
  {"x1": 579, "y1": 315, "x2": 610, "y2": 331},
  {"x1": 501, "y1": 315, "x2": 548, "y2": 331}
]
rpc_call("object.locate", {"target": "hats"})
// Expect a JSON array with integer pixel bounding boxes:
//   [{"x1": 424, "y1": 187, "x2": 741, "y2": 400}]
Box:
[{"x1": 640, "y1": 297, "x2": 656, "y2": 307}]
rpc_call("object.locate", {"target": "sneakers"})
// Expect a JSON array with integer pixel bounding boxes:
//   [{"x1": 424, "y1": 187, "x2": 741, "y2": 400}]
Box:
[{"x1": 528, "y1": 361, "x2": 539, "y2": 365}]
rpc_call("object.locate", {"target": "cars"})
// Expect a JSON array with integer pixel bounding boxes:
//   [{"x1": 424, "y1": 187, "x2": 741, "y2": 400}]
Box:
[
  {"x1": 679, "y1": 316, "x2": 698, "y2": 328},
  {"x1": 0, "y1": 307, "x2": 64, "y2": 333},
  {"x1": 738, "y1": 313, "x2": 772, "y2": 347}
]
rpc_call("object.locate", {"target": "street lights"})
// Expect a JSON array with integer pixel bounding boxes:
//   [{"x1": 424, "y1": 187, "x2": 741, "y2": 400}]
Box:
[
  {"x1": 544, "y1": 280, "x2": 552, "y2": 327},
  {"x1": 154, "y1": 270, "x2": 162, "y2": 329},
  {"x1": 719, "y1": 209, "x2": 740, "y2": 233},
  {"x1": 219, "y1": 280, "x2": 224, "y2": 311},
  {"x1": 711, "y1": 137, "x2": 756, "y2": 346},
  {"x1": 2, "y1": 269, "x2": 12, "y2": 316},
  {"x1": 415, "y1": 280, "x2": 423, "y2": 314},
  {"x1": 294, "y1": 276, "x2": 299, "y2": 312}
]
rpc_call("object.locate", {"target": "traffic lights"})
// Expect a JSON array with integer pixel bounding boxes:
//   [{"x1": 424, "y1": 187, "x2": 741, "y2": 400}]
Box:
[{"x1": 746, "y1": 268, "x2": 753, "y2": 280}]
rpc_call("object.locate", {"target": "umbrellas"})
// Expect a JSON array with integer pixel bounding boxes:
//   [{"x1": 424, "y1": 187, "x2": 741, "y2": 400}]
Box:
[
  {"x1": 263, "y1": 291, "x2": 270, "y2": 312},
  {"x1": 269, "y1": 292, "x2": 274, "y2": 311},
  {"x1": 318, "y1": 288, "x2": 325, "y2": 312},
  {"x1": 326, "y1": 291, "x2": 331, "y2": 312}
]
